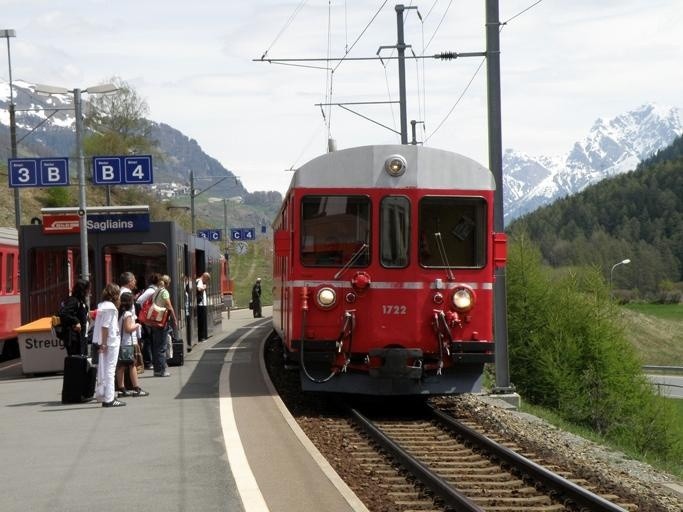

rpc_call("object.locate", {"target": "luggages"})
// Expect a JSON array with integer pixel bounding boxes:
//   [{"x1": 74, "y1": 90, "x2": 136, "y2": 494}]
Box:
[
  {"x1": 167, "y1": 338, "x2": 184, "y2": 365},
  {"x1": 61, "y1": 325, "x2": 97, "y2": 404}
]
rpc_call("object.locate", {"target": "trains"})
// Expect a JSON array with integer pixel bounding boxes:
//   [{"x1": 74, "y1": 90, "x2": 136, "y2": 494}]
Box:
[
  {"x1": 272, "y1": 145, "x2": 506, "y2": 395},
  {"x1": 0, "y1": 225, "x2": 235, "y2": 342}
]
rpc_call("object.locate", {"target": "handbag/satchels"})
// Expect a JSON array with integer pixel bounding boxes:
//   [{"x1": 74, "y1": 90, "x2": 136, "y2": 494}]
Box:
[
  {"x1": 249, "y1": 300, "x2": 254, "y2": 309},
  {"x1": 118, "y1": 345, "x2": 134, "y2": 363},
  {"x1": 138, "y1": 299, "x2": 168, "y2": 329}
]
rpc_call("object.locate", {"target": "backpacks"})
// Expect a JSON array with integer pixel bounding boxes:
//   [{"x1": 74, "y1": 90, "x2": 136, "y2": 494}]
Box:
[
  {"x1": 51, "y1": 296, "x2": 84, "y2": 339},
  {"x1": 195, "y1": 281, "x2": 203, "y2": 305}
]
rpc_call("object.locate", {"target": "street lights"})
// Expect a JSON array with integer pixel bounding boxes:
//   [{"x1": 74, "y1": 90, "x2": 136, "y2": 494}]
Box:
[
  {"x1": 207, "y1": 196, "x2": 241, "y2": 318},
  {"x1": 610, "y1": 258, "x2": 630, "y2": 308},
  {"x1": 34, "y1": 82, "x2": 118, "y2": 306},
  {"x1": 0, "y1": 29, "x2": 19, "y2": 229}
]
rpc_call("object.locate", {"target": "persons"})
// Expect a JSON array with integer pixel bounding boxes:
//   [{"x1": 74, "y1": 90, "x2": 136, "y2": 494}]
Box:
[
  {"x1": 183, "y1": 275, "x2": 190, "y2": 316},
  {"x1": 314, "y1": 236, "x2": 344, "y2": 266},
  {"x1": 195, "y1": 271, "x2": 210, "y2": 343},
  {"x1": 251, "y1": 278, "x2": 264, "y2": 319},
  {"x1": 53, "y1": 270, "x2": 177, "y2": 408}
]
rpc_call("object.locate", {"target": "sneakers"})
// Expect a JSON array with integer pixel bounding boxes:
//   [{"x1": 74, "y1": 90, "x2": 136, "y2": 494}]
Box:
[
  {"x1": 118, "y1": 387, "x2": 149, "y2": 397},
  {"x1": 102, "y1": 398, "x2": 126, "y2": 407}
]
